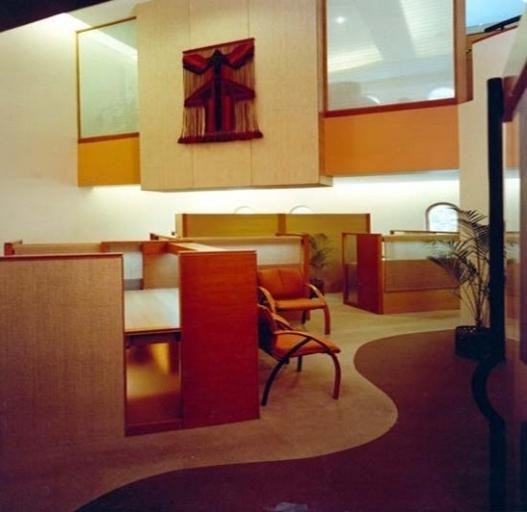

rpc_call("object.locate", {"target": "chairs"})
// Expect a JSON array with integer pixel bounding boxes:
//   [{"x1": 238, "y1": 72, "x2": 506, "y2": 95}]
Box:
[
  {"x1": 257, "y1": 263, "x2": 331, "y2": 335},
  {"x1": 256, "y1": 285, "x2": 342, "y2": 406}
]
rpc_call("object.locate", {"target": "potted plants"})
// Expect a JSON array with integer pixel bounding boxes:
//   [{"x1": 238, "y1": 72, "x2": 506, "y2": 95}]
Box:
[
  {"x1": 424, "y1": 210, "x2": 509, "y2": 360},
  {"x1": 302, "y1": 232, "x2": 334, "y2": 297}
]
